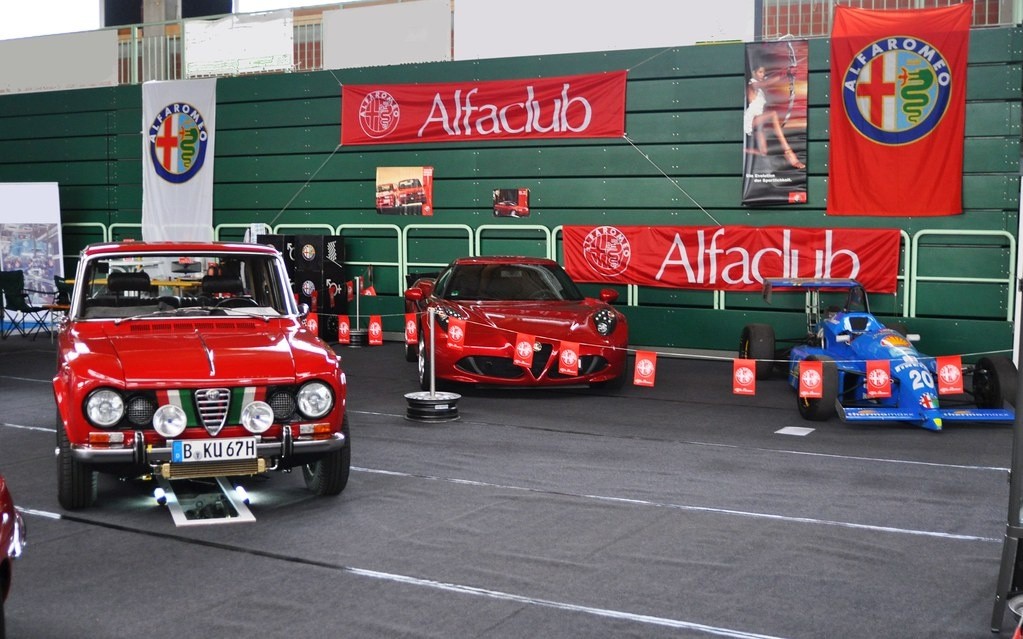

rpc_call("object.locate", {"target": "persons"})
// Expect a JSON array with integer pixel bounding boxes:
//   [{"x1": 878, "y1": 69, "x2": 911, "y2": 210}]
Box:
[{"x1": 744, "y1": 63, "x2": 806, "y2": 168}]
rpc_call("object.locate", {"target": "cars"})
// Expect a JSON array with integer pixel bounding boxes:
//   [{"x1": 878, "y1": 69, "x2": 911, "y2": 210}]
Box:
[
  {"x1": 376, "y1": 184, "x2": 398, "y2": 207},
  {"x1": 396, "y1": 180, "x2": 428, "y2": 205}
]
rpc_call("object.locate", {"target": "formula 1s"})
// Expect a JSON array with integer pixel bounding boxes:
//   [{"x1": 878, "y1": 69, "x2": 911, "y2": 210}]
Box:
[{"x1": 740, "y1": 278, "x2": 1016, "y2": 432}]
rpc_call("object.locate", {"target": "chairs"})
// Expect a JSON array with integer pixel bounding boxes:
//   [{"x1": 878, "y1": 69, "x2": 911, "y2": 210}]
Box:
[
  {"x1": 823, "y1": 306, "x2": 842, "y2": 320},
  {"x1": 201, "y1": 274, "x2": 253, "y2": 307},
  {"x1": 0, "y1": 270, "x2": 73, "y2": 342},
  {"x1": 108, "y1": 272, "x2": 151, "y2": 307}
]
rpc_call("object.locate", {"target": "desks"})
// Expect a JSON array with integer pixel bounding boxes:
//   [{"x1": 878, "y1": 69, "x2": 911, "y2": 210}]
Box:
[{"x1": 42, "y1": 304, "x2": 71, "y2": 343}]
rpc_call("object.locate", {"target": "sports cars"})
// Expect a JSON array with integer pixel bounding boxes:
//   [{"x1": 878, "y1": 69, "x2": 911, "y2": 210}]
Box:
[
  {"x1": 404, "y1": 257, "x2": 630, "y2": 396},
  {"x1": 494, "y1": 201, "x2": 530, "y2": 218}
]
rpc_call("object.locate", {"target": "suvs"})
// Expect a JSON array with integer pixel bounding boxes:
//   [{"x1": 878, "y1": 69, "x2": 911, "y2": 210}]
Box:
[{"x1": 49, "y1": 239, "x2": 351, "y2": 513}]
